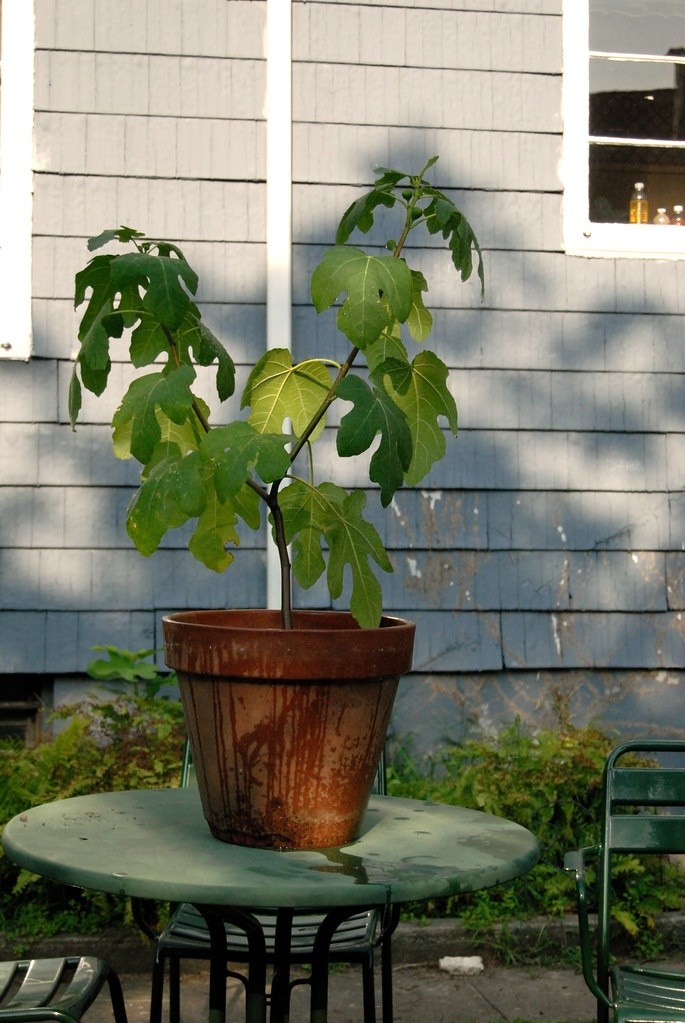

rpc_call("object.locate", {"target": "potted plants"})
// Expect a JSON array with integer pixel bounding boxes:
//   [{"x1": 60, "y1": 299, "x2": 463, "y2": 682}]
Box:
[{"x1": 63, "y1": 155, "x2": 487, "y2": 853}]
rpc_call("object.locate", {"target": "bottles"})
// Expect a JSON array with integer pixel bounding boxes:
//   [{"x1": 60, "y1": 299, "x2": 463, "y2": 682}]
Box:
[
  {"x1": 629, "y1": 182, "x2": 649, "y2": 224},
  {"x1": 653, "y1": 208, "x2": 670, "y2": 224},
  {"x1": 669, "y1": 205, "x2": 685, "y2": 226}
]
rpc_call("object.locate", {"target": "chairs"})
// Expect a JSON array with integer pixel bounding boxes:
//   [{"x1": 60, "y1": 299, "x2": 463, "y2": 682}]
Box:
[
  {"x1": 149, "y1": 735, "x2": 393, "y2": 1023},
  {"x1": 562, "y1": 739, "x2": 685, "y2": 1023},
  {"x1": 0, "y1": 954, "x2": 129, "y2": 1023}
]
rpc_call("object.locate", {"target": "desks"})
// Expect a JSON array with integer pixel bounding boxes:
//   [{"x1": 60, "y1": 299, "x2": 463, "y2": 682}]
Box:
[{"x1": 0, "y1": 787, "x2": 537, "y2": 1023}]
